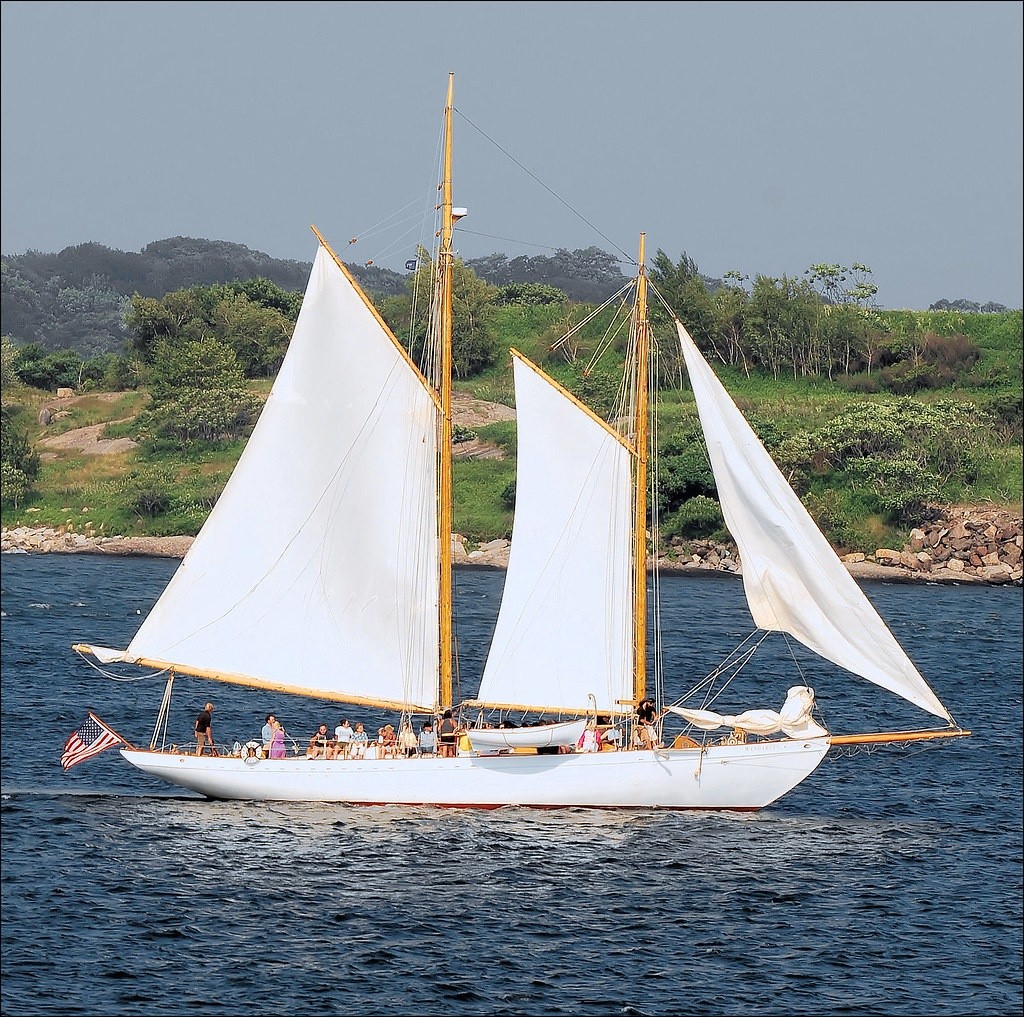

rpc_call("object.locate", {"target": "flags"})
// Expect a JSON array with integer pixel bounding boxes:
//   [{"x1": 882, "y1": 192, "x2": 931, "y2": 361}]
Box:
[{"x1": 60, "y1": 717, "x2": 123, "y2": 773}]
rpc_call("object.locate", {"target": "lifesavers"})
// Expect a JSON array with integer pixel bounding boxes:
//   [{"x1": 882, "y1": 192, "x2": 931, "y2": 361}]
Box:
[{"x1": 239, "y1": 741, "x2": 263, "y2": 764}]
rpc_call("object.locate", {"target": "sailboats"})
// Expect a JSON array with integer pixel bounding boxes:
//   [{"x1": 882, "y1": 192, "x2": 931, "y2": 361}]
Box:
[{"x1": 71, "y1": 72, "x2": 973, "y2": 812}]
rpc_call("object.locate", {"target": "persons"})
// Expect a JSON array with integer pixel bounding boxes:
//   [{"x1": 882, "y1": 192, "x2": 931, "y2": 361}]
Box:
[
  {"x1": 261, "y1": 714, "x2": 287, "y2": 759},
  {"x1": 195, "y1": 702, "x2": 212, "y2": 756},
  {"x1": 312, "y1": 719, "x2": 368, "y2": 759},
  {"x1": 637, "y1": 698, "x2": 658, "y2": 750},
  {"x1": 578, "y1": 716, "x2": 610, "y2": 752},
  {"x1": 402, "y1": 721, "x2": 438, "y2": 757},
  {"x1": 378, "y1": 724, "x2": 397, "y2": 746},
  {"x1": 439, "y1": 709, "x2": 457, "y2": 745}
]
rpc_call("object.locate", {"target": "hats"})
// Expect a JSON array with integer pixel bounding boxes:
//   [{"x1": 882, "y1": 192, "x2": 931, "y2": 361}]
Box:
[
  {"x1": 422, "y1": 722, "x2": 432, "y2": 728},
  {"x1": 585, "y1": 720, "x2": 597, "y2": 729},
  {"x1": 384, "y1": 725, "x2": 395, "y2": 732}
]
